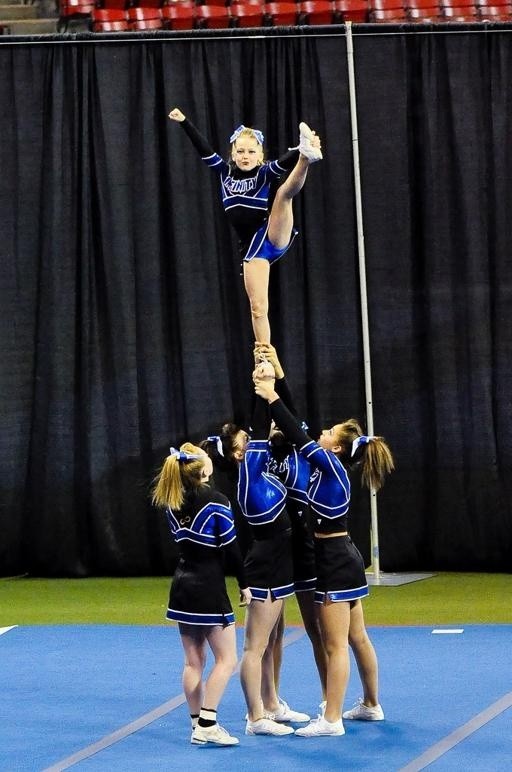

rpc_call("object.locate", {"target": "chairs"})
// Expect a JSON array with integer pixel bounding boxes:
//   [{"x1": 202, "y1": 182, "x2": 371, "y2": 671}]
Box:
[{"x1": 56, "y1": 0, "x2": 511, "y2": 32}]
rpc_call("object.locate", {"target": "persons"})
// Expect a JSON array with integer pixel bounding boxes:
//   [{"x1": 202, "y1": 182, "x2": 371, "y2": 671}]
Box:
[
  {"x1": 164, "y1": 105, "x2": 325, "y2": 380},
  {"x1": 255, "y1": 382, "x2": 394, "y2": 736},
  {"x1": 199, "y1": 364, "x2": 309, "y2": 736},
  {"x1": 244, "y1": 343, "x2": 328, "y2": 722},
  {"x1": 152, "y1": 443, "x2": 252, "y2": 745}
]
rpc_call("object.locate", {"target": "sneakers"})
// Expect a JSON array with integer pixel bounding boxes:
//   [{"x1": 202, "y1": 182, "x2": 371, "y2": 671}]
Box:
[
  {"x1": 288, "y1": 122, "x2": 323, "y2": 163},
  {"x1": 191, "y1": 696, "x2": 384, "y2": 746}
]
